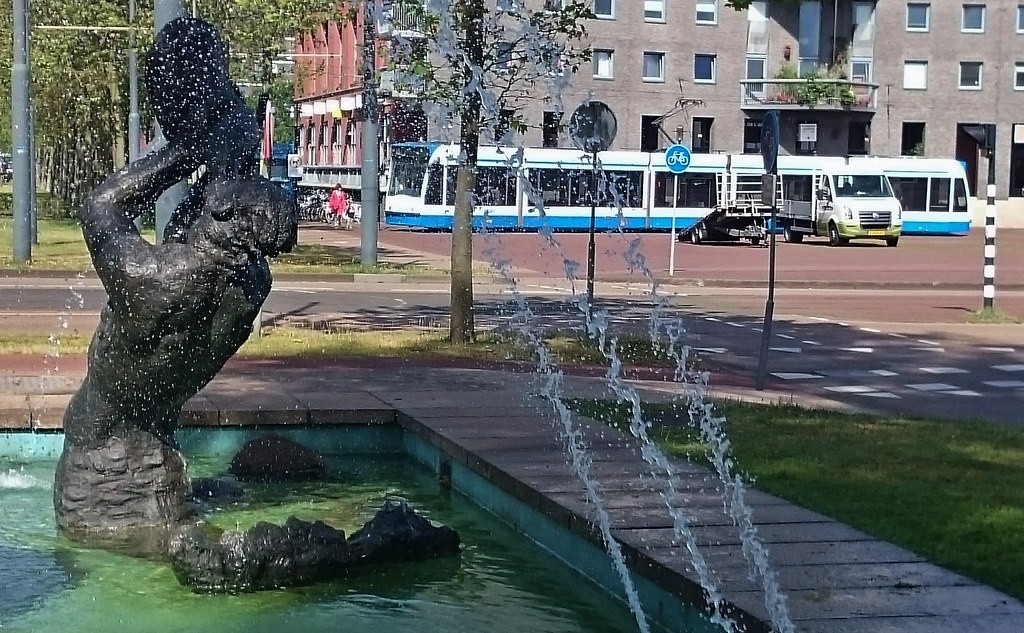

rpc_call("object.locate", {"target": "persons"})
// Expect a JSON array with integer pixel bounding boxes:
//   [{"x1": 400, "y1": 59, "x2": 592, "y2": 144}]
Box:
[
  {"x1": 52, "y1": 76, "x2": 300, "y2": 560},
  {"x1": 344, "y1": 202, "x2": 356, "y2": 230},
  {"x1": 329, "y1": 182, "x2": 347, "y2": 228}
]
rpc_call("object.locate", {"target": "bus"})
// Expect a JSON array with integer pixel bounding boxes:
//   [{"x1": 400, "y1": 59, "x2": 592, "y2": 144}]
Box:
[{"x1": 382, "y1": 142, "x2": 973, "y2": 233}]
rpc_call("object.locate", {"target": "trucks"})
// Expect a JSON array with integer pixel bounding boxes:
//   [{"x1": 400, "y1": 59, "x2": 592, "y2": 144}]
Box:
[{"x1": 782, "y1": 168, "x2": 903, "y2": 247}]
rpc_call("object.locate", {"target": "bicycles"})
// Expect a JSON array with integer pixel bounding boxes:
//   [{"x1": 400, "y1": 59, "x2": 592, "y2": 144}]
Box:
[{"x1": 295, "y1": 190, "x2": 342, "y2": 224}]
